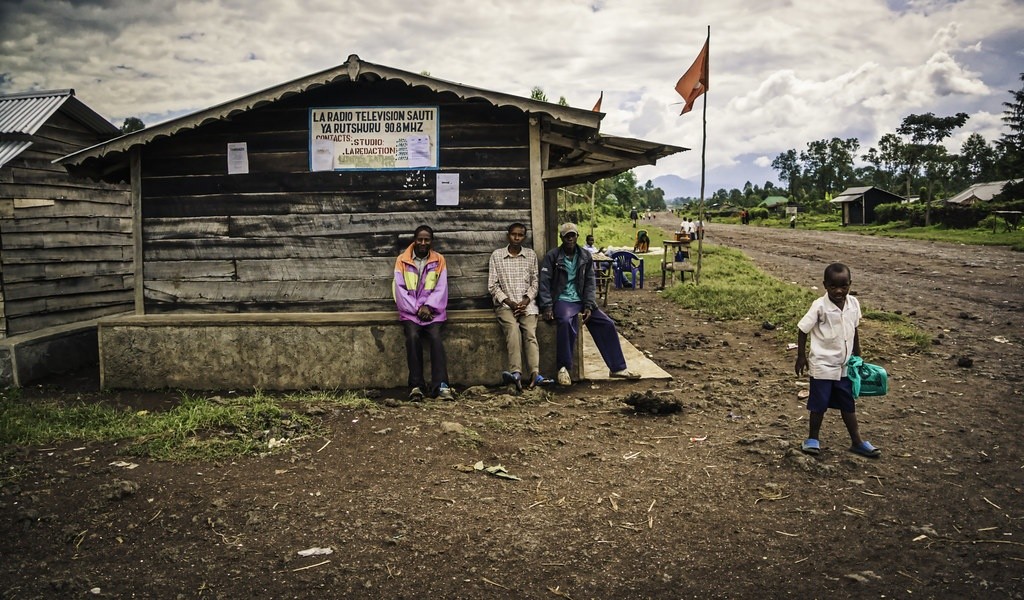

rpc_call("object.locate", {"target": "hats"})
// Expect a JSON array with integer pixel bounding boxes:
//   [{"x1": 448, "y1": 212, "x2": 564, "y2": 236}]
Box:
[{"x1": 560, "y1": 222, "x2": 578, "y2": 237}]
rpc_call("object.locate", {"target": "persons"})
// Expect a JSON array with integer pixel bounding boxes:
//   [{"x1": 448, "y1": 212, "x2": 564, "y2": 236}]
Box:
[
  {"x1": 688, "y1": 218, "x2": 695, "y2": 240},
  {"x1": 630, "y1": 207, "x2": 638, "y2": 228},
  {"x1": 487, "y1": 223, "x2": 554, "y2": 392},
  {"x1": 790, "y1": 214, "x2": 795, "y2": 228},
  {"x1": 794, "y1": 264, "x2": 881, "y2": 457},
  {"x1": 538, "y1": 223, "x2": 641, "y2": 386},
  {"x1": 695, "y1": 217, "x2": 704, "y2": 239},
  {"x1": 582, "y1": 235, "x2": 633, "y2": 288},
  {"x1": 393, "y1": 225, "x2": 454, "y2": 402},
  {"x1": 741, "y1": 208, "x2": 749, "y2": 224},
  {"x1": 680, "y1": 217, "x2": 691, "y2": 232}
]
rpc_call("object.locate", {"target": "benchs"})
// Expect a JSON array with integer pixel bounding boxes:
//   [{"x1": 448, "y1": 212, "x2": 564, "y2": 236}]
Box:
[
  {"x1": 96, "y1": 309, "x2": 584, "y2": 395},
  {"x1": 0, "y1": 311, "x2": 136, "y2": 388}
]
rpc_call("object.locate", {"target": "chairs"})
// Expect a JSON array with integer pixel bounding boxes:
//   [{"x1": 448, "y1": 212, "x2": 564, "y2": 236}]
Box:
[{"x1": 611, "y1": 252, "x2": 644, "y2": 291}]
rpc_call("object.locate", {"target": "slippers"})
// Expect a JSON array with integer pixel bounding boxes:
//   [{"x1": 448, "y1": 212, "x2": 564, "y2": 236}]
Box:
[
  {"x1": 801, "y1": 438, "x2": 820, "y2": 453},
  {"x1": 502, "y1": 371, "x2": 523, "y2": 390},
  {"x1": 850, "y1": 441, "x2": 881, "y2": 456},
  {"x1": 530, "y1": 375, "x2": 555, "y2": 385}
]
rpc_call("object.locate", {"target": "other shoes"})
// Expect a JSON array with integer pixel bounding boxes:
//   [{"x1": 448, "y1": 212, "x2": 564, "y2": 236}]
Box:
[
  {"x1": 609, "y1": 369, "x2": 641, "y2": 379},
  {"x1": 409, "y1": 387, "x2": 425, "y2": 400},
  {"x1": 558, "y1": 367, "x2": 572, "y2": 386},
  {"x1": 435, "y1": 383, "x2": 454, "y2": 401},
  {"x1": 624, "y1": 281, "x2": 633, "y2": 288}
]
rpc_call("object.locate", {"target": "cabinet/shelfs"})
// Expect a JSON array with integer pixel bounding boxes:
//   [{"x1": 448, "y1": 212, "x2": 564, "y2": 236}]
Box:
[{"x1": 660, "y1": 240, "x2": 695, "y2": 287}]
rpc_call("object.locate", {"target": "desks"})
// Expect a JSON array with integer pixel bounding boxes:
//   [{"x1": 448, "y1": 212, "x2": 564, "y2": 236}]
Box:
[
  {"x1": 587, "y1": 252, "x2": 615, "y2": 308},
  {"x1": 991, "y1": 210, "x2": 1022, "y2": 234}
]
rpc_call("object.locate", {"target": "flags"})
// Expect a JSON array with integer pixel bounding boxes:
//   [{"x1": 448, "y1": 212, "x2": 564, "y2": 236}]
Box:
[{"x1": 674, "y1": 36, "x2": 709, "y2": 116}]
